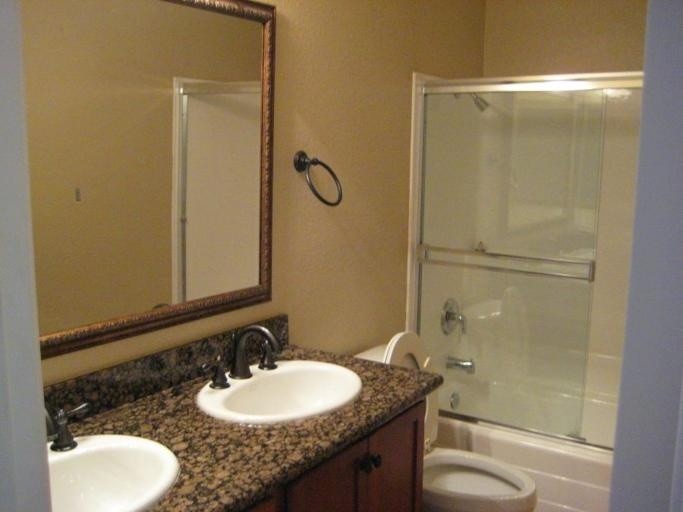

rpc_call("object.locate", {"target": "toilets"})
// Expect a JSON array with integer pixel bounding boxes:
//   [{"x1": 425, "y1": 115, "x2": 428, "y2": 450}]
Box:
[
  {"x1": 355, "y1": 330, "x2": 535, "y2": 512},
  {"x1": 466, "y1": 287, "x2": 583, "y2": 431}
]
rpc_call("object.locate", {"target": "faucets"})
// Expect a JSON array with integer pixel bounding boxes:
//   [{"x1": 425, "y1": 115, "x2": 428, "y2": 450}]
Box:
[
  {"x1": 42, "y1": 407, "x2": 59, "y2": 440},
  {"x1": 233, "y1": 322, "x2": 281, "y2": 378}
]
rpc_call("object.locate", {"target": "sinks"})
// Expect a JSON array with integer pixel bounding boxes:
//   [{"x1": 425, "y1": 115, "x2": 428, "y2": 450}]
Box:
[
  {"x1": 196, "y1": 359, "x2": 361, "y2": 425},
  {"x1": 48, "y1": 434, "x2": 179, "y2": 512}
]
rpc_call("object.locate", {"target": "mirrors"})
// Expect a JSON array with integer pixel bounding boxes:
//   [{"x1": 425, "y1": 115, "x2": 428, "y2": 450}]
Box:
[{"x1": 20, "y1": 1, "x2": 276, "y2": 361}]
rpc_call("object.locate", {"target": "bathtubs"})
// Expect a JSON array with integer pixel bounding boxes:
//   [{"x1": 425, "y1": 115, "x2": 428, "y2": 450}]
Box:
[{"x1": 429, "y1": 368, "x2": 619, "y2": 510}]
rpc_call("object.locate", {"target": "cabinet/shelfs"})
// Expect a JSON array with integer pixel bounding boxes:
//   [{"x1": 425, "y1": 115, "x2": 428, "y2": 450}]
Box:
[{"x1": 245, "y1": 399, "x2": 426, "y2": 512}]
